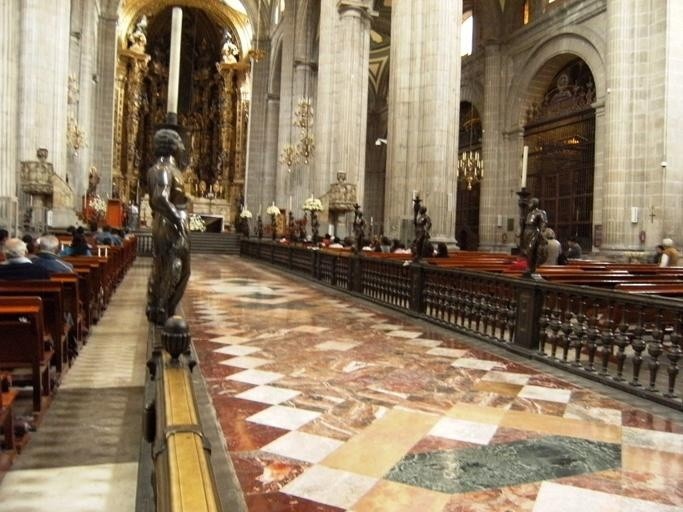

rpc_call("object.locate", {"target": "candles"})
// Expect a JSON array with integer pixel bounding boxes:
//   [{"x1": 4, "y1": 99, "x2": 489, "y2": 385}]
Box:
[
  {"x1": 193, "y1": 183, "x2": 223, "y2": 193},
  {"x1": 289, "y1": 196, "x2": 292, "y2": 211},
  {"x1": 521, "y1": 144, "x2": 529, "y2": 189}
]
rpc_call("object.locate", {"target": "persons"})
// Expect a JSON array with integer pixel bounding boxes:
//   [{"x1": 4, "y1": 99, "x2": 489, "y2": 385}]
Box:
[
  {"x1": 653, "y1": 245, "x2": 664, "y2": 262},
  {"x1": 312, "y1": 215, "x2": 320, "y2": 234},
  {"x1": 658, "y1": 238, "x2": 679, "y2": 267},
  {"x1": 145, "y1": 128, "x2": 190, "y2": 325},
  {"x1": 288, "y1": 211, "x2": 295, "y2": 240},
  {"x1": 315, "y1": 232, "x2": 451, "y2": 258},
  {"x1": 522, "y1": 198, "x2": 548, "y2": 278},
  {"x1": 353, "y1": 211, "x2": 366, "y2": 252},
  {"x1": 0, "y1": 223, "x2": 136, "y2": 358},
  {"x1": 271, "y1": 214, "x2": 276, "y2": 240},
  {"x1": 504, "y1": 229, "x2": 582, "y2": 271},
  {"x1": 415, "y1": 207, "x2": 431, "y2": 260},
  {"x1": 257, "y1": 216, "x2": 263, "y2": 239}
]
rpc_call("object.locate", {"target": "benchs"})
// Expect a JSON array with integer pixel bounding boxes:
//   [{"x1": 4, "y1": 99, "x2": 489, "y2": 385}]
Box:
[
  {"x1": 320, "y1": 249, "x2": 683, "y2": 320},
  {"x1": 0, "y1": 229, "x2": 141, "y2": 476}
]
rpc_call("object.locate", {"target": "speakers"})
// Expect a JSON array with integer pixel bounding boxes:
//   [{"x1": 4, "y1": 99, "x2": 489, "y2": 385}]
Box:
[
  {"x1": 631, "y1": 207, "x2": 639, "y2": 224},
  {"x1": 497, "y1": 214, "x2": 502, "y2": 227}
]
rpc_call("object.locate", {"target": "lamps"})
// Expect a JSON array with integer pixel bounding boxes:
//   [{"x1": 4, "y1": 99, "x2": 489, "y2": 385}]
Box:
[
  {"x1": 453, "y1": 9, "x2": 486, "y2": 192},
  {"x1": 276, "y1": 13, "x2": 317, "y2": 174}
]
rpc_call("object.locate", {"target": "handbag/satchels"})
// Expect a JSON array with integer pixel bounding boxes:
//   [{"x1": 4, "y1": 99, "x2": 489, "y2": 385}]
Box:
[{"x1": 558, "y1": 253, "x2": 568, "y2": 265}]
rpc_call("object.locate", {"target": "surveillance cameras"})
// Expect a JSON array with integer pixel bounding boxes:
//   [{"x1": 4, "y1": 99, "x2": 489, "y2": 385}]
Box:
[{"x1": 375, "y1": 140, "x2": 381, "y2": 147}]
